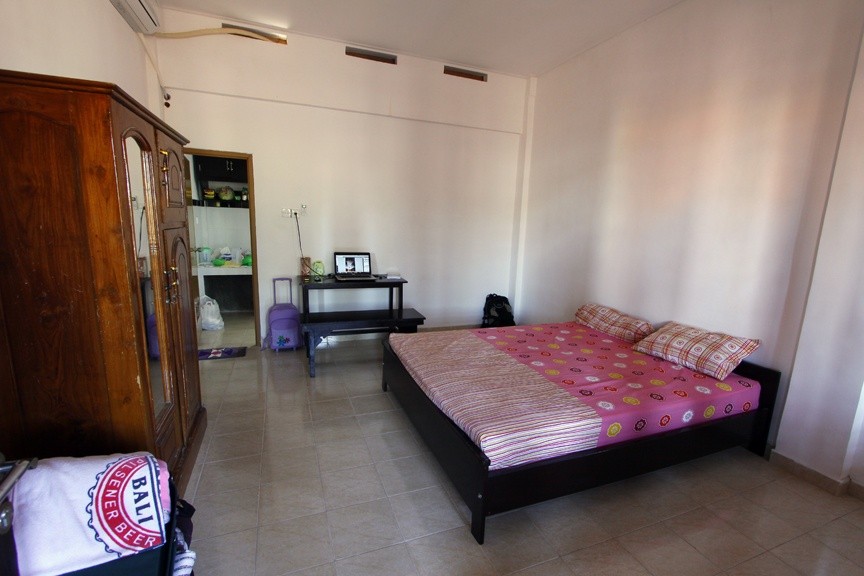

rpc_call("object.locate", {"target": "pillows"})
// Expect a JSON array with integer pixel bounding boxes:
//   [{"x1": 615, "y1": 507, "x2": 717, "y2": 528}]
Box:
[
  {"x1": 575, "y1": 303, "x2": 656, "y2": 343},
  {"x1": 632, "y1": 321, "x2": 761, "y2": 383}
]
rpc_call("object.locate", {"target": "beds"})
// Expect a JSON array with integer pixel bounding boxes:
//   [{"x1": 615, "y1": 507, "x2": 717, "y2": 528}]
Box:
[{"x1": 382, "y1": 322, "x2": 781, "y2": 545}]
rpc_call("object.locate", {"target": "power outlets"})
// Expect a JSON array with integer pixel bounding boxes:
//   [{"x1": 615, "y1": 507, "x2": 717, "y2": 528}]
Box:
[{"x1": 291, "y1": 209, "x2": 299, "y2": 217}]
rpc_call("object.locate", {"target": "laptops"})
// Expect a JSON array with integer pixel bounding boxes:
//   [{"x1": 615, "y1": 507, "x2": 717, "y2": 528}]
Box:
[{"x1": 334, "y1": 252, "x2": 376, "y2": 281}]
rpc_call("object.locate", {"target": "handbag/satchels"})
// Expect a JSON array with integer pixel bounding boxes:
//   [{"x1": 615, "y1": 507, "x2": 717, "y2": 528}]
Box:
[{"x1": 199, "y1": 296, "x2": 224, "y2": 331}]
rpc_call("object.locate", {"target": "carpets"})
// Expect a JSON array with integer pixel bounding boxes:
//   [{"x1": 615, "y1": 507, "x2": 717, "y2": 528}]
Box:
[{"x1": 198, "y1": 346, "x2": 246, "y2": 360}]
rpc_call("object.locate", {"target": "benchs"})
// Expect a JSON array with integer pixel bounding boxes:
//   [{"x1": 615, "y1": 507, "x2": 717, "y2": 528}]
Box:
[{"x1": 297, "y1": 308, "x2": 426, "y2": 378}]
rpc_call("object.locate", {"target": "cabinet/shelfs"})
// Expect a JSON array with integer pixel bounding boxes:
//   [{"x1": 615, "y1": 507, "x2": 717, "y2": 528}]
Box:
[
  {"x1": 0, "y1": 69, "x2": 206, "y2": 498},
  {"x1": 193, "y1": 155, "x2": 248, "y2": 183}
]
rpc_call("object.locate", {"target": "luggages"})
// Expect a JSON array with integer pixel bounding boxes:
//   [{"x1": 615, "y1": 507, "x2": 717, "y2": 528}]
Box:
[{"x1": 269, "y1": 277, "x2": 303, "y2": 352}]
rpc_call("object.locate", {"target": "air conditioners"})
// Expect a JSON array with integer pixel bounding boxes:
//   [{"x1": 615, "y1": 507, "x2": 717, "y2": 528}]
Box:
[{"x1": 108, "y1": 0, "x2": 160, "y2": 34}]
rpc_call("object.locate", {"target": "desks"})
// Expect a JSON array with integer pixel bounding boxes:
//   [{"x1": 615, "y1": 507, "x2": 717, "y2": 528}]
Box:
[{"x1": 298, "y1": 274, "x2": 408, "y2": 358}]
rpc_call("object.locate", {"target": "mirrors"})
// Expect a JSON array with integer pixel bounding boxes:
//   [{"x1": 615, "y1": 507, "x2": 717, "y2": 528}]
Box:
[{"x1": 123, "y1": 136, "x2": 167, "y2": 419}]
